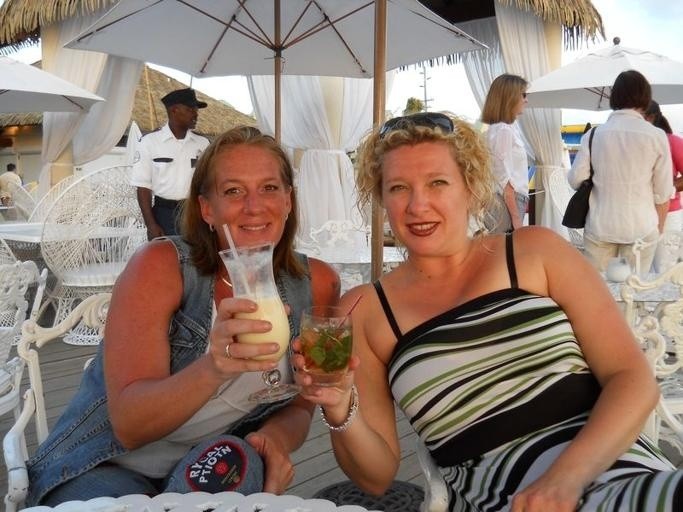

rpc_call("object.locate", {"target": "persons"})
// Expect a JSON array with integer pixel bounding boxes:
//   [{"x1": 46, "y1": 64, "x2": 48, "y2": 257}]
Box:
[
  {"x1": 479, "y1": 73, "x2": 530, "y2": 234},
  {"x1": 129, "y1": 89, "x2": 212, "y2": 243},
  {"x1": 0, "y1": 164, "x2": 22, "y2": 206},
  {"x1": 568, "y1": 68, "x2": 683, "y2": 282},
  {"x1": 11, "y1": 124, "x2": 341, "y2": 506},
  {"x1": 292, "y1": 111, "x2": 683, "y2": 512}
]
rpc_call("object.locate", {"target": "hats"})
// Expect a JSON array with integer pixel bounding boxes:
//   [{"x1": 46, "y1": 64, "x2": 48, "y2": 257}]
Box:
[{"x1": 161, "y1": 90, "x2": 207, "y2": 108}]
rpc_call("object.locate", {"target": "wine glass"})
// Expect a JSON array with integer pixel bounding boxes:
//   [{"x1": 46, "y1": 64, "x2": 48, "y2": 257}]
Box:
[{"x1": 218, "y1": 241, "x2": 302, "y2": 403}]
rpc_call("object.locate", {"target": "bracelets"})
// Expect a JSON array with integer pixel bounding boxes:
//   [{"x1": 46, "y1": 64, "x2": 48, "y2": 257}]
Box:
[{"x1": 319, "y1": 386, "x2": 359, "y2": 433}]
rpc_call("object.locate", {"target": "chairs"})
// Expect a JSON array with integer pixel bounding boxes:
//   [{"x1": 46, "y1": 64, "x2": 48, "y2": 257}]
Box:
[
  {"x1": 294, "y1": 226, "x2": 320, "y2": 260},
  {"x1": 564, "y1": 225, "x2": 681, "y2": 466},
  {"x1": 311, "y1": 220, "x2": 373, "y2": 248},
  {"x1": 1, "y1": 145, "x2": 148, "y2": 345},
  {"x1": 1, "y1": 239, "x2": 48, "y2": 462},
  {"x1": 2, "y1": 294, "x2": 113, "y2": 512}
]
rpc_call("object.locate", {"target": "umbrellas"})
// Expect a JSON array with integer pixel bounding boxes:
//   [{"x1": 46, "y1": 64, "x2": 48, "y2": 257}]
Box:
[
  {"x1": 0, "y1": 53, "x2": 104, "y2": 115},
  {"x1": 62, "y1": 0, "x2": 491, "y2": 146},
  {"x1": 526, "y1": 37, "x2": 683, "y2": 110}
]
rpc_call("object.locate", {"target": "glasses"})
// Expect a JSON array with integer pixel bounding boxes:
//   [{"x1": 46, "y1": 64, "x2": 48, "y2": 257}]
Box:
[{"x1": 378, "y1": 113, "x2": 453, "y2": 138}]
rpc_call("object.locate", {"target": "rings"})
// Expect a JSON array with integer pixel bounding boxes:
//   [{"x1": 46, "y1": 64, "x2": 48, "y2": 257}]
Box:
[{"x1": 225, "y1": 343, "x2": 232, "y2": 358}]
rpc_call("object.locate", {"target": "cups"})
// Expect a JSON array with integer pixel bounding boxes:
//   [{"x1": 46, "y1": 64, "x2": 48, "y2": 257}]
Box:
[
  {"x1": 605, "y1": 257, "x2": 630, "y2": 281},
  {"x1": 298, "y1": 304, "x2": 352, "y2": 388}
]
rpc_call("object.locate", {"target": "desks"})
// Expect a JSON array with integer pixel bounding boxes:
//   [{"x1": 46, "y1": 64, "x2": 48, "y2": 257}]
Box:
[
  {"x1": 317, "y1": 246, "x2": 412, "y2": 287},
  {"x1": 9, "y1": 490, "x2": 378, "y2": 511}
]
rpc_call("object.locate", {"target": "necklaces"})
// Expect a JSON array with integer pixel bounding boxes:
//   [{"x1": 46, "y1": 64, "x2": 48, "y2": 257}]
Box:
[{"x1": 218, "y1": 271, "x2": 233, "y2": 288}]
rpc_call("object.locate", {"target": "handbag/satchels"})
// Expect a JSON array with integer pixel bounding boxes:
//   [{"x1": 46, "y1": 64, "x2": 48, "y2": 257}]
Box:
[{"x1": 562, "y1": 180, "x2": 593, "y2": 228}]
